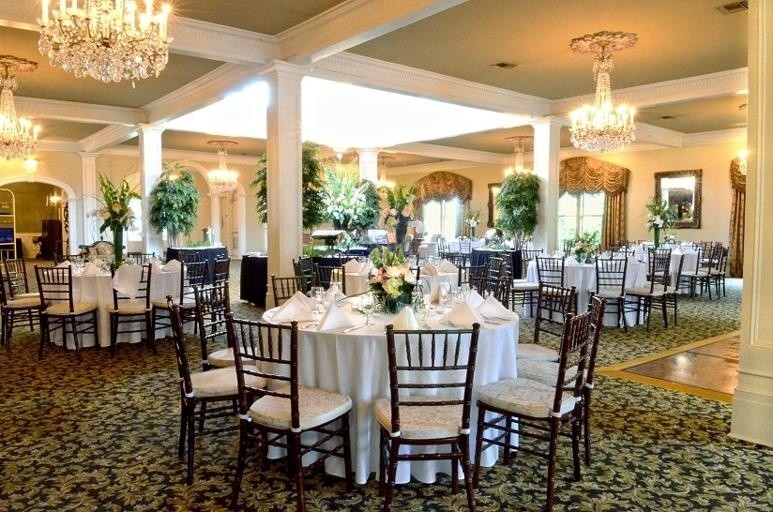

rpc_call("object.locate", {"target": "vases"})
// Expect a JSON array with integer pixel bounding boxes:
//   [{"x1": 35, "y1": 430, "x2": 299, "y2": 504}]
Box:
[
  {"x1": 113, "y1": 225, "x2": 122, "y2": 265},
  {"x1": 471, "y1": 227, "x2": 475, "y2": 241},
  {"x1": 654, "y1": 224, "x2": 660, "y2": 249},
  {"x1": 396, "y1": 222, "x2": 407, "y2": 244}
]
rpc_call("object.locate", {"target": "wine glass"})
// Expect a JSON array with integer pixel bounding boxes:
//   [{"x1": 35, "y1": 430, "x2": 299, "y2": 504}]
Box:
[
  {"x1": 436, "y1": 281, "x2": 468, "y2": 315},
  {"x1": 208, "y1": 226, "x2": 215, "y2": 247},
  {"x1": 574, "y1": 240, "x2": 692, "y2": 264},
  {"x1": 311, "y1": 287, "x2": 323, "y2": 314},
  {"x1": 68, "y1": 255, "x2": 163, "y2": 272},
  {"x1": 330, "y1": 282, "x2": 343, "y2": 307},
  {"x1": 360, "y1": 295, "x2": 376, "y2": 327}
]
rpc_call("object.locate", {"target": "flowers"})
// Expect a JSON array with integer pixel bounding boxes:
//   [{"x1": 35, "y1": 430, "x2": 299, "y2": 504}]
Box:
[
  {"x1": 82, "y1": 170, "x2": 143, "y2": 262},
  {"x1": 464, "y1": 208, "x2": 480, "y2": 227},
  {"x1": 317, "y1": 171, "x2": 416, "y2": 231},
  {"x1": 647, "y1": 200, "x2": 678, "y2": 248}
]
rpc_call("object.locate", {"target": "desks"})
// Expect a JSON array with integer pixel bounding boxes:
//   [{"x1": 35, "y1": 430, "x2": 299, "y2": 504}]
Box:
[
  {"x1": 261, "y1": 295, "x2": 524, "y2": 484},
  {"x1": 619, "y1": 242, "x2": 707, "y2": 294},
  {"x1": 526, "y1": 254, "x2": 651, "y2": 330},
  {"x1": 41, "y1": 255, "x2": 188, "y2": 346}
]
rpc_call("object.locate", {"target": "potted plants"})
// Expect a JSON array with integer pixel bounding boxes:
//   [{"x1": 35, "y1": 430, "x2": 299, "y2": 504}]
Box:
[{"x1": 495, "y1": 171, "x2": 542, "y2": 279}]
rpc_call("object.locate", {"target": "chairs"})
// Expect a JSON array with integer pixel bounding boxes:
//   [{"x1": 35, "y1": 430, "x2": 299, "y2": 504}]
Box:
[
  {"x1": 1, "y1": 257, "x2": 45, "y2": 350},
  {"x1": 161, "y1": 292, "x2": 267, "y2": 481},
  {"x1": 513, "y1": 282, "x2": 608, "y2": 460},
  {"x1": 272, "y1": 245, "x2": 514, "y2": 307},
  {"x1": 229, "y1": 314, "x2": 354, "y2": 512},
  {"x1": 534, "y1": 255, "x2": 580, "y2": 324},
  {"x1": 482, "y1": 310, "x2": 596, "y2": 511},
  {"x1": 627, "y1": 246, "x2": 682, "y2": 331},
  {"x1": 681, "y1": 240, "x2": 729, "y2": 302},
  {"x1": 376, "y1": 320, "x2": 480, "y2": 509},
  {"x1": 107, "y1": 264, "x2": 157, "y2": 355},
  {"x1": 585, "y1": 255, "x2": 628, "y2": 331},
  {"x1": 158, "y1": 247, "x2": 240, "y2": 356},
  {"x1": 32, "y1": 261, "x2": 102, "y2": 357}
]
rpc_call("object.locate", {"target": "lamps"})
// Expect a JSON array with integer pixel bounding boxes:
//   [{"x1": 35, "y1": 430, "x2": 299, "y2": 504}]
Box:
[
  {"x1": 207, "y1": 141, "x2": 239, "y2": 192},
  {"x1": 738, "y1": 104, "x2": 748, "y2": 176},
  {"x1": 569, "y1": 31, "x2": 638, "y2": 152},
  {"x1": 376, "y1": 155, "x2": 398, "y2": 187},
  {"x1": 0, "y1": 55, "x2": 38, "y2": 161},
  {"x1": 36, "y1": 0, "x2": 173, "y2": 89},
  {"x1": 504, "y1": 136, "x2": 534, "y2": 176}
]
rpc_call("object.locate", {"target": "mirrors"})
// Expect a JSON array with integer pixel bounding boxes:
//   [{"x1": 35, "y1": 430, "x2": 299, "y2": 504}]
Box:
[{"x1": 655, "y1": 169, "x2": 702, "y2": 229}]
